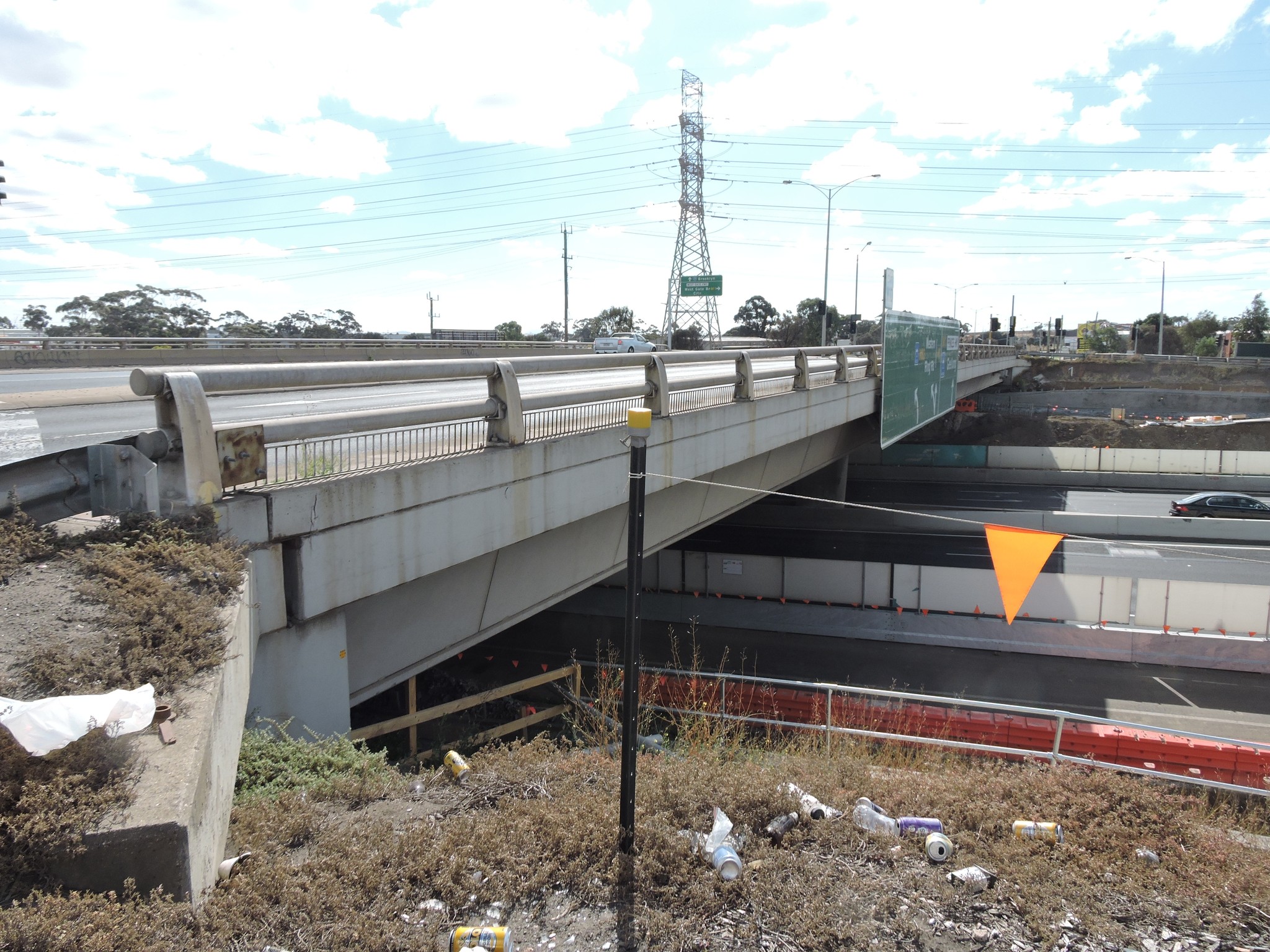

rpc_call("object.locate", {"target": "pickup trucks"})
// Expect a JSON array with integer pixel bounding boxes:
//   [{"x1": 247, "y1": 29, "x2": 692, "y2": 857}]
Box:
[{"x1": 593, "y1": 331, "x2": 658, "y2": 354}]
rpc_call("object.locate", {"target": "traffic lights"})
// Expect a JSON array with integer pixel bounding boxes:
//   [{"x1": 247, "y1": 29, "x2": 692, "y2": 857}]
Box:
[
  {"x1": 1215, "y1": 334, "x2": 1222, "y2": 348},
  {"x1": 0, "y1": 160, "x2": 7, "y2": 205},
  {"x1": 1008, "y1": 326, "x2": 1015, "y2": 337},
  {"x1": 991, "y1": 318, "x2": 1001, "y2": 331},
  {"x1": 850, "y1": 322, "x2": 856, "y2": 334},
  {"x1": 1224, "y1": 339, "x2": 1229, "y2": 346},
  {"x1": 819, "y1": 300, "x2": 826, "y2": 315}
]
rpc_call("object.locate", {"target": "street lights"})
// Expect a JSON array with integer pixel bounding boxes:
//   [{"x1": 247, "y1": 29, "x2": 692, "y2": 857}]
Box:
[
  {"x1": 934, "y1": 283, "x2": 978, "y2": 320},
  {"x1": 1006, "y1": 314, "x2": 1022, "y2": 345},
  {"x1": 1034, "y1": 322, "x2": 1042, "y2": 351},
  {"x1": 844, "y1": 242, "x2": 873, "y2": 355},
  {"x1": 782, "y1": 174, "x2": 882, "y2": 357},
  {"x1": 1125, "y1": 256, "x2": 1166, "y2": 356},
  {"x1": 982, "y1": 314, "x2": 999, "y2": 344},
  {"x1": 961, "y1": 306, "x2": 993, "y2": 350}
]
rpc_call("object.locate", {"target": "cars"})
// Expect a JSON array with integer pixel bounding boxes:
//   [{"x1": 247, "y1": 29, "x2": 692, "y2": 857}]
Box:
[{"x1": 1169, "y1": 492, "x2": 1270, "y2": 520}]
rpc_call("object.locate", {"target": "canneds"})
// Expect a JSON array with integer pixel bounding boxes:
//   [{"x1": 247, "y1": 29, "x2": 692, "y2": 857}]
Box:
[
  {"x1": 443, "y1": 750, "x2": 471, "y2": 781},
  {"x1": 925, "y1": 833, "x2": 953, "y2": 861},
  {"x1": 893, "y1": 817, "x2": 944, "y2": 839},
  {"x1": 855, "y1": 797, "x2": 885, "y2": 814},
  {"x1": 711, "y1": 845, "x2": 742, "y2": 880},
  {"x1": 1012, "y1": 820, "x2": 1064, "y2": 844},
  {"x1": 448, "y1": 925, "x2": 513, "y2": 952}
]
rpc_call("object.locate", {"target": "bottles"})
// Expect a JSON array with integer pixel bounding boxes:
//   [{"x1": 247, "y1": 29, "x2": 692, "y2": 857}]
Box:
[
  {"x1": 763, "y1": 812, "x2": 799, "y2": 848},
  {"x1": 777, "y1": 782, "x2": 824, "y2": 820},
  {"x1": 856, "y1": 797, "x2": 888, "y2": 816},
  {"x1": 852, "y1": 805, "x2": 896, "y2": 835}
]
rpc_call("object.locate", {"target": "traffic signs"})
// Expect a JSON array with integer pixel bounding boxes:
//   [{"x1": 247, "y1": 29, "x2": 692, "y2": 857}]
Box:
[
  {"x1": 880, "y1": 309, "x2": 960, "y2": 449},
  {"x1": 680, "y1": 274, "x2": 723, "y2": 296}
]
